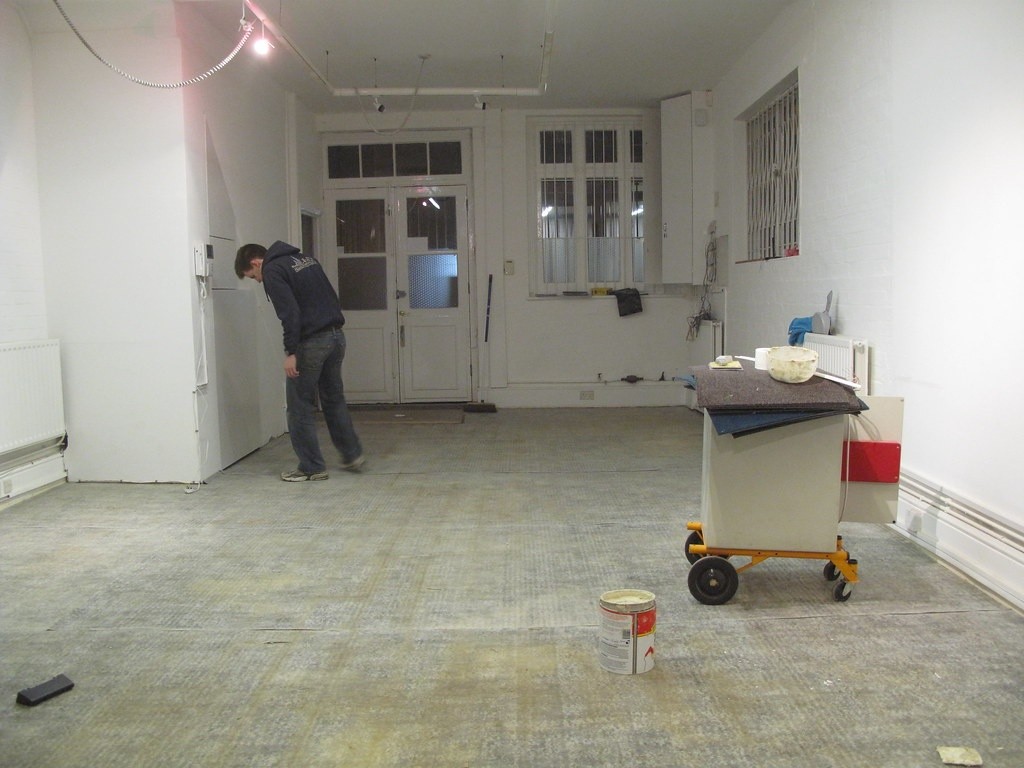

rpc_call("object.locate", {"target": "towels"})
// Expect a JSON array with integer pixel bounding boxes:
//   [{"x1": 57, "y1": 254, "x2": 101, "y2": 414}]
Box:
[{"x1": 608, "y1": 287, "x2": 644, "y2": 319}]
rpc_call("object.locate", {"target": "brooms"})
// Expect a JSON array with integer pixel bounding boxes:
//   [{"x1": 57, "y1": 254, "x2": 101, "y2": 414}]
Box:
[{"x1": 463, "y1": 274, "x2": 499, "y2": 414}]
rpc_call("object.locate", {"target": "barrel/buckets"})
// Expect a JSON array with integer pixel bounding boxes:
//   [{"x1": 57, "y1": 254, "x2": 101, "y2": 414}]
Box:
[{"x1": 598, "y1": 589, "x2": 657, "y2": 675}]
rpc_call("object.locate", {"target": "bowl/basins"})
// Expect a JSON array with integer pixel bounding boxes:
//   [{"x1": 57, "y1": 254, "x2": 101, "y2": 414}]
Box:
[{"x1": 766, "y1": 345, "x2": 819, "y2": 384}]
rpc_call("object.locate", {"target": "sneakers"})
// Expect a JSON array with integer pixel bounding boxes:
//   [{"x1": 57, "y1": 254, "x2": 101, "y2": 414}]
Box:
[
  {"x1": 338, "y1": 451, "x2": 365, "y2": 472},
  {"x1": 281, "y1": 466, "x2": 330, "y2": 482}
]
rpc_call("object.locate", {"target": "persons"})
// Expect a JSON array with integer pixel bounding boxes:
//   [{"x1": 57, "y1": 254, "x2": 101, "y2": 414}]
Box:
[{"x1": 233, "y1": 238, "x2": 365, "y2": 485}]
